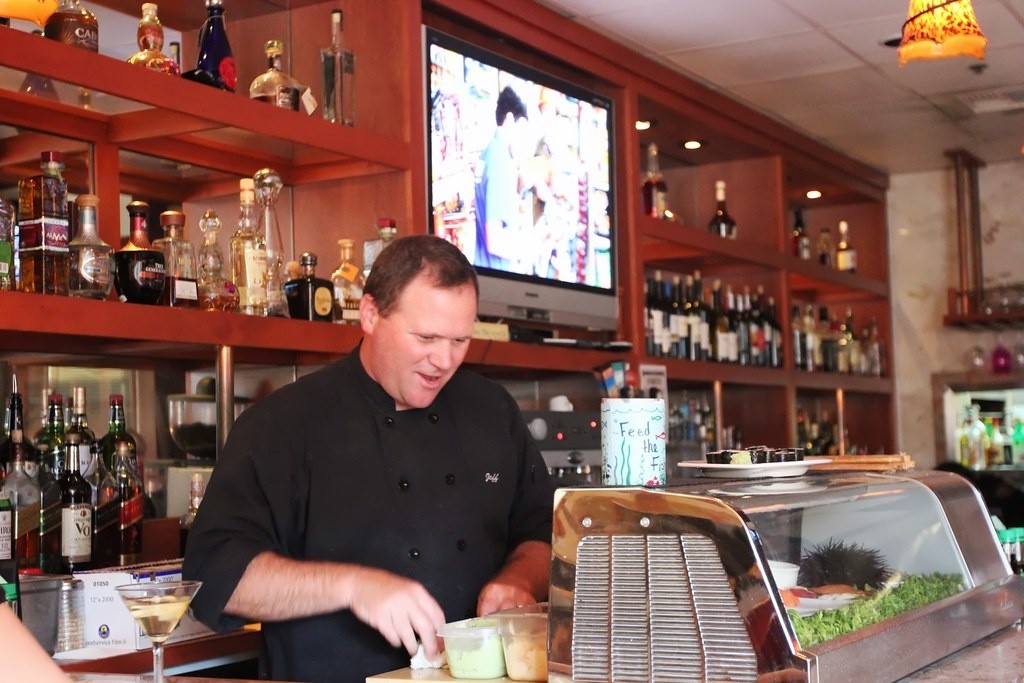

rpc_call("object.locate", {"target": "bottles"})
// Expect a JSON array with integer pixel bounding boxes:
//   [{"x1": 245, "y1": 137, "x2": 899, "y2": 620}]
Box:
[
  {"x1": 791, "y1": 305, "x2": 885, "y2": 378},
  {"x1": 668, "y1": 389, "x2": 714, "y2": 442},
  {"x1": 0, "y1": 373, "x2": 143, "y2": 574},
  {"x1": 646, "y1": 268, "x2": 783, "y2": 368},
  {"x1": 18, "y1": 0, "x2": 358, "y2": 128},
  {"x1": 2, "y1": 151, "x2": 398, "y2": 326},
  {"x1": 956, "y1": 404, "x2": 1024, "y2": 471},
  {"x1": 709, "y1": 180, "x2": 738, "y2": 238},
  {"x1": 54, "y1": 579, "x2": 87, "y2": 653},
  {"x1": 818, "y1": 222, "x2": 858, "y2": 273},
  {"x1": 178, "y1": 472, "x2": 206, "y2": 558},
  {"x1": 788, "y1": 205, "x2": 811, "y2": 261},
  {"x1": 797, "y1": 405, "x2": 871, "y2": 456},
  {"x1": 643, "y1": 142, "x2": 670, "y2": 221},
  {"x1": 997, "y1": 528, "x2": 1024, "y2": 576},
  {"x1": 722, "y1": 423, "x2": 743, "y2": 449}
]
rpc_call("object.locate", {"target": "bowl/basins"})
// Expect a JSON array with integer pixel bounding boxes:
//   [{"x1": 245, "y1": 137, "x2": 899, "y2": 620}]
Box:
[
  {"x1": 436, "y1": 618, "x2": 507, "y2": 680},
  {"x1": 165, "y1": 394, "x2": 249, "y2": 461},
  {"x1": 486, "y1": 602, "x2": 554, "y2": 683}
]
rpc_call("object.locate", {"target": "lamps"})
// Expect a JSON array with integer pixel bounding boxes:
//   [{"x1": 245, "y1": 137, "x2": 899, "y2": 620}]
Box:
[{"x1": 898, "y1": 0, "x2": 989, "y2": 66}]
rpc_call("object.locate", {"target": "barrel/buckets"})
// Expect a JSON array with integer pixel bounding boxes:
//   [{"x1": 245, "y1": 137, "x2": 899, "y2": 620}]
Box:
[{"x1": 767, "y1": 560, "x2": 800, "y2": 590}]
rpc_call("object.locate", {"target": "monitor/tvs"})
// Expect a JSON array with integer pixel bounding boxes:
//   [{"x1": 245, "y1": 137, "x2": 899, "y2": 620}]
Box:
[{"x1": 421, "y1": 23, "x2": 620, "y2": 332}]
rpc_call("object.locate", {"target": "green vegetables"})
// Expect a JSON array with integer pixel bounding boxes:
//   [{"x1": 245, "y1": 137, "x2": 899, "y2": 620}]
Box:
[{"x1": 788, "y1": 573, "x2": 966, "y2": 649}]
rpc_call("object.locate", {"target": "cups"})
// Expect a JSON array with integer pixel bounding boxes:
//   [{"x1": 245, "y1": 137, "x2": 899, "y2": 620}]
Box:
[{"x1": 600, "y1": 396, "x2": 666, "y2": 487}]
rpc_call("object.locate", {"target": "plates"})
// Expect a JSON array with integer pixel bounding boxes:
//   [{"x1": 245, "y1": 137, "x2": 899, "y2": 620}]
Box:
[
  {"x1": 786, "y1": 598, "x2": 849, "y2": 618},
  {"x1": 676, "y1": 458, "x2": 833, "y2": 478}
]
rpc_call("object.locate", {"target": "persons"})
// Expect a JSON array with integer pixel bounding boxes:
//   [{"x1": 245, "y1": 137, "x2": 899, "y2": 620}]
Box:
[
  {"x1": 181, "y1": 235, "x2": 555, "y2": 683},
  {"x1": 473, "y1": 86, "x2": 557, "y2": 277}
]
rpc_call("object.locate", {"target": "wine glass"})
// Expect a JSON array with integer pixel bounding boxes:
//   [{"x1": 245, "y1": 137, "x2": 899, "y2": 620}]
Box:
[
  {"x1": 116, "y1": 581, "x2": 203, "y2": 683},
  {"x1": 964, "y1": 322, "x2": 1024, "y2": 377}
]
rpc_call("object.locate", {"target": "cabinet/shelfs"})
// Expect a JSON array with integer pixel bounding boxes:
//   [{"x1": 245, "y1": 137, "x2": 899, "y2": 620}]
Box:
[
  {"x1": 0, "y1": 0, "x2": 420, "y2": 602},
  {"x1": 635, "y1": 90, "x2": 897, "y2": 481}
]
